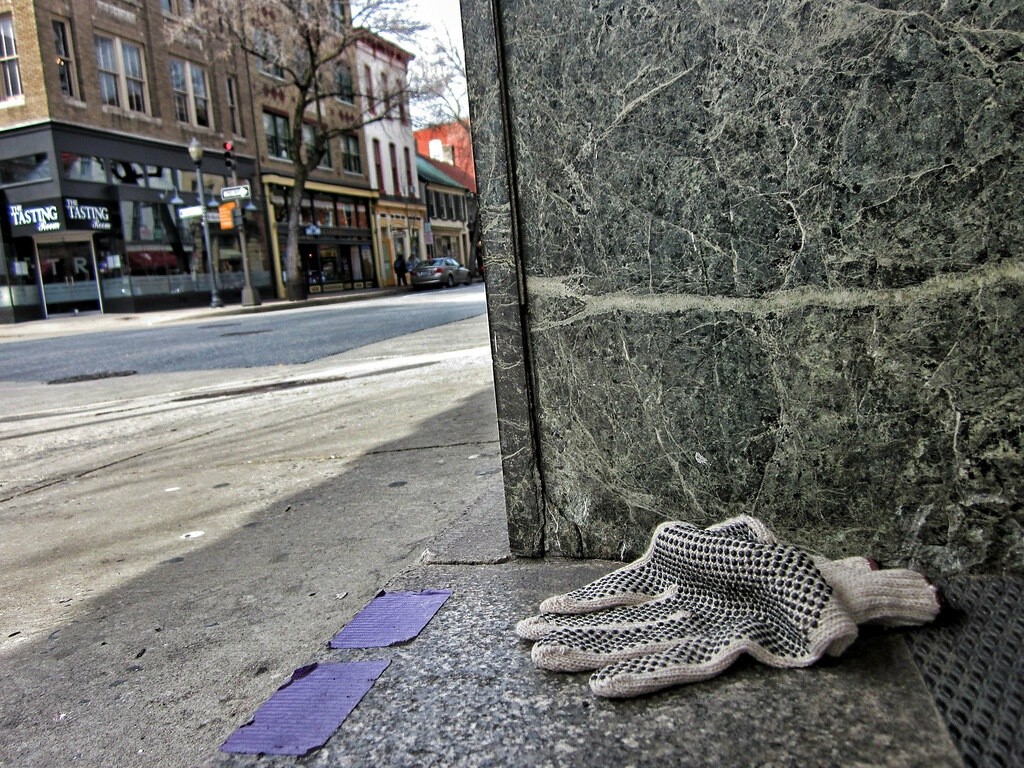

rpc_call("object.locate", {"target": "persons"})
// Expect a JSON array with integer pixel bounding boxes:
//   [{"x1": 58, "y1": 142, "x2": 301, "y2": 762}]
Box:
[{"x1": 394, "y1": 252, "x2": 420, "y2": 286}]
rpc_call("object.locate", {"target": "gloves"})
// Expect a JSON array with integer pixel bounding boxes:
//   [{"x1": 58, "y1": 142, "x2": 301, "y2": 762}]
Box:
[{"x1": 514, "y1": 511, "x2": 942, "y2": 700}]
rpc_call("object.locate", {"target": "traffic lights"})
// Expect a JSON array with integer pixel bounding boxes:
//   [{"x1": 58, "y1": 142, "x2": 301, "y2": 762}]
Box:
[{"x1": 223, "y1": 141, "x2": 238, "y2": 172}]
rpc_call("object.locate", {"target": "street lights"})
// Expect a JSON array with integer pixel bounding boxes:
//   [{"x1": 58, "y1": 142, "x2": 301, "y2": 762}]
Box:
[{"x1": 188, "y1": 137, "x2": 223, "y2": 307}]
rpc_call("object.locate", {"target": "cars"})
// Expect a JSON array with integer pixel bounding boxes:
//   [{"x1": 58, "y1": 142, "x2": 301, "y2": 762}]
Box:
[{"x1": 409, "y1": 257, "x2": 473, "y2": 289}]
